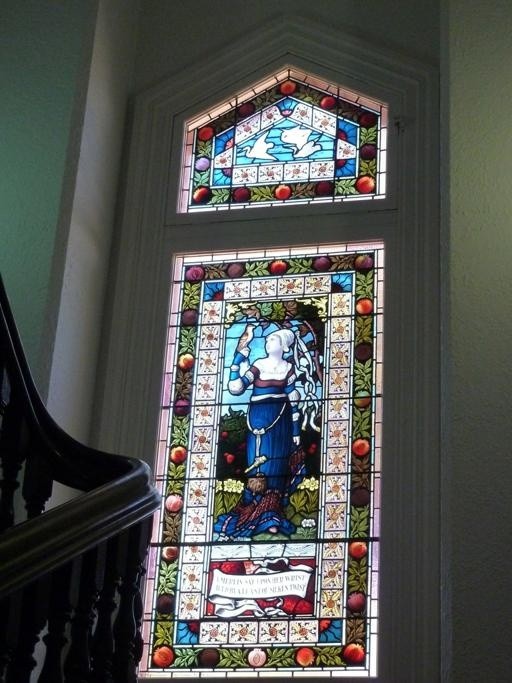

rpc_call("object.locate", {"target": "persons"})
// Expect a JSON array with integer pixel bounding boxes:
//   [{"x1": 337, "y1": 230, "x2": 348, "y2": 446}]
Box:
[{"x1": 214, "y1": 328, "x2": 308, "y2": 537}]
